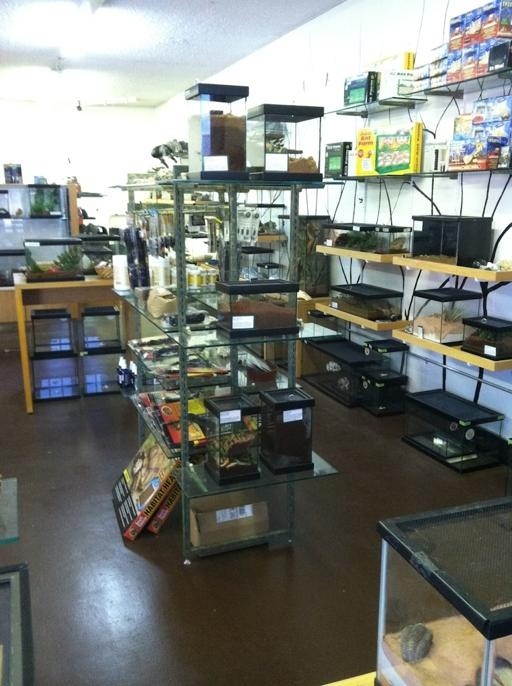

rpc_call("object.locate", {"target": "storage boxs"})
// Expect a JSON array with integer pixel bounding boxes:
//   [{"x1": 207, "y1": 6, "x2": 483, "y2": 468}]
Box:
[{"x1": 187, "y1": 488, "x2": 270, "y2": 547}]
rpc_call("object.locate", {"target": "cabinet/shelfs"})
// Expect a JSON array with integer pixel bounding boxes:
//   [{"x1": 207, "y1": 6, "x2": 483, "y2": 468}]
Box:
[
  {"x1": 299, "y1": 68, "x2": 512, "y2": 474},
  {"x1": 0, "y1": 180, "x2": 81, "y2": 324},
  {"x1": 105, "y1": 180, "x2": 349, "y2": 561}
]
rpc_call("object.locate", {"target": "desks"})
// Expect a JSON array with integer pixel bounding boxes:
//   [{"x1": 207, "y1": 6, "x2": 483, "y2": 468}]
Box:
[{"x1": 12, "y1": 272, "x2": 136, "y2": 414}]
